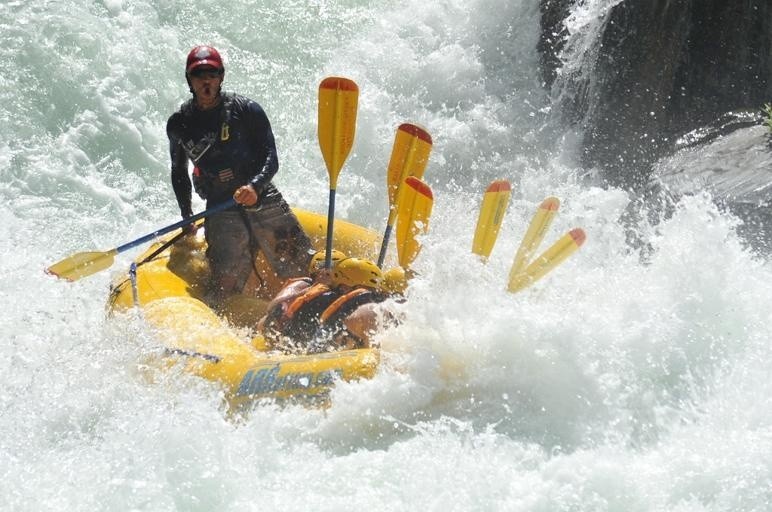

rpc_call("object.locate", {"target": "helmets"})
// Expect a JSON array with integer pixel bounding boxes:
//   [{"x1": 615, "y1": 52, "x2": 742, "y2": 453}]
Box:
[{"x1": 310, "y1": 249, "x2": 407, "y2": 296}]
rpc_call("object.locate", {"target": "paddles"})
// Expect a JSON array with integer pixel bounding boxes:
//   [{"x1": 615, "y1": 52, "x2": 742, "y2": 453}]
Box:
[
  {"x1": 43, "y1": 189, "x2": 241, "y2": 281},
  {"x1": 509, "y1": 199, "x2": 586, "y2": 293},
  {"x1": 377, "y1": 125, "x2": 434, "y2": 269},
  {"x1": 318, "y1": 77, "x2": 358, "y2": 270},
  {"x1": 473, "y1": 180, "x2": 511, "y2": 263}
]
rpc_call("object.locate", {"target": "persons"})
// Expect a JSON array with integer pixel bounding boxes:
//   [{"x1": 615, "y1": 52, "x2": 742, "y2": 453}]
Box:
[
  {"x1": 252, "y1": 246, "x2": 409, "y2": 356},
  {"x1": 165, "y1": 45, "x2": 315, "y2": 305}
]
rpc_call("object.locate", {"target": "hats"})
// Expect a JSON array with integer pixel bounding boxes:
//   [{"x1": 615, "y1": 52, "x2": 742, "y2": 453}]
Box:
[{"x1": 186, "y1": 45, "x2": 221, "y2": 72}]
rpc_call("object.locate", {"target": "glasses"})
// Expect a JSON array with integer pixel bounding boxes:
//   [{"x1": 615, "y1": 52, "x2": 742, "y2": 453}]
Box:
[{"x1": 192, "y1": 67, "x2": 217, "y2": 79}]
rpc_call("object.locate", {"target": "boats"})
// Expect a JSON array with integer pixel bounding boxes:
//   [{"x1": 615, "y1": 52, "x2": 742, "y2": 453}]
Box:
[{"x1": 106, "y1": 207, "x2": 476, "y2": 433}]
